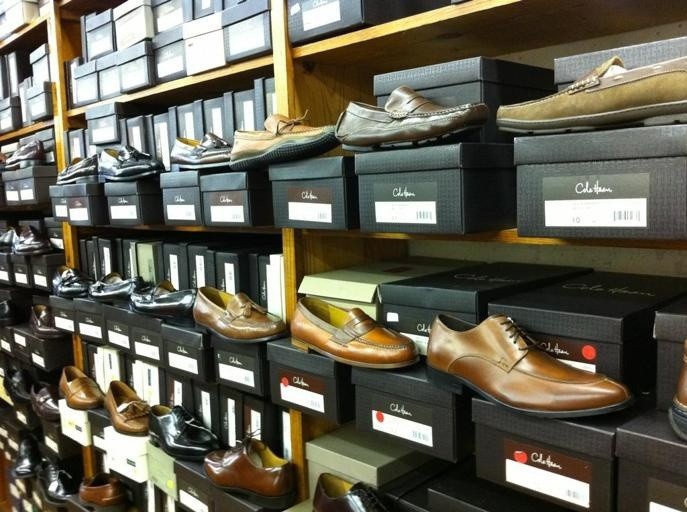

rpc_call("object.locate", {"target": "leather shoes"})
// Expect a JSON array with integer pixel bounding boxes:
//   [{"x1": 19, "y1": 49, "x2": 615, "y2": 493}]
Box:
[
  {"x1": 495, "y1": 53, "x2": 687, "y2": 138},
  {"x1": 1, "y1": 227, "x2": 19, "y2": 253},
  {"x1": 129, "y1": 280, "x2": 197, "y2": 328},
  {"x1": 333, "y1": 84, "x2": 499, "y2": 150},
  {"x1": 104, "y1": 380, "x2": 151, "y2": 436},
  {"x1": 29, "y1": 304, "x2": 62, "y2": 339},
  {"x1": 426, "y1": 311, "x2": 631, "y2": 419},
  {"x1": 668, "y1": 354, "x2": 687, "y2": 441},
  {"x1": 51, "y1": 266, "x2": 88, "y2": 298},
  {"x1": 4, "y1": 358, "x2": 32, "y2": 404},
  {"x1": 291, "y1": 297, "x2": 420, "y2": 369},
  {"x1": 96, "y1": 144, "x2": 164, "y2": 184},
  {"x1": 5, "y1": 140, "x2": 48, "y2": 170},
  {"x1": 72, "y1": 470, "x2": 131, "y2": 510},
  {"x1": 204, "y1": 427, "x2": 303, "y2": 511},
  {"x1": 229, "y1": 109, "x2": 341, "y2": 166},
  {"x1": 1, "y1": 298, "x2": 18, "y2": 326},
  {"x1": 87, "y1": 273, "x2": 144, "y2": 309},
  {"x1": 36, "y1": 455, "x2": 73, "y2": 506},
  {"x1": 59, "y1": 366, "x2": 104, "y2": 411},
  {"x1": 193, "y1": 286, "x2": 285, "y2": 344},
  {"x1": 30, "y1": 381, "x2": 61, "y2": 421},
  {"x1": 149, "y1": 405, "x2": 220, "y2": 458},
  {"x1": 311, "y1": 469, "x2": 404, "y2": 512},
  {"x1": 11, "y1": 434, "x2": 41, "y2": 479},
  {"x1": 55, "y1": 153, "x2": 100, "y2": 186},
  {"x1": 167, "y1": 131, "x2": 234, "y2": 171},
  {"x1": 14, "y1": 226, "x2": 55, "y2": 255}
]
